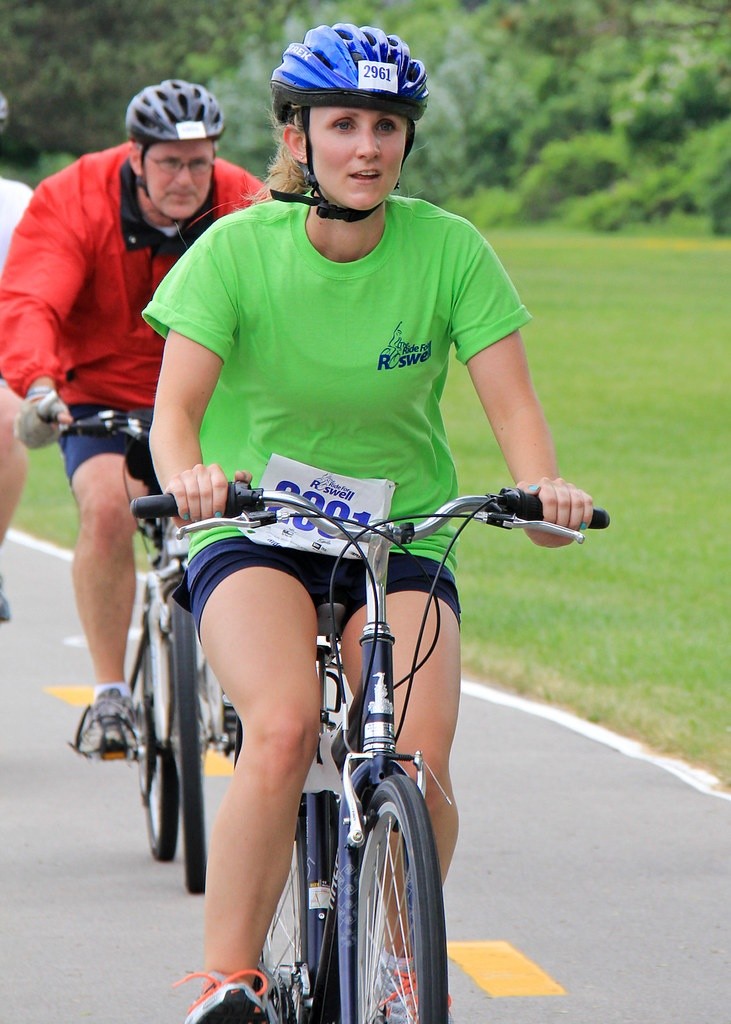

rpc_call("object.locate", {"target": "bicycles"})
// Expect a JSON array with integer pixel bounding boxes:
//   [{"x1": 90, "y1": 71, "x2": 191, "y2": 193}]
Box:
[
  {"x1": 128, "y1": 474, "x2": 610, "y2": 1023},
  {"x1": 60, "y1": 408, "x2": 241, "y2": 895}
]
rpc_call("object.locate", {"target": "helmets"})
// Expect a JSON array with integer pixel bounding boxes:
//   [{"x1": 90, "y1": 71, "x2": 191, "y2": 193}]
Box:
[
  {"x1": 267, "y1": 20, "x2": 429, "y2": 122},
  {"x1": 123, "y1": 74, "x2": 227, "y2": 142}
]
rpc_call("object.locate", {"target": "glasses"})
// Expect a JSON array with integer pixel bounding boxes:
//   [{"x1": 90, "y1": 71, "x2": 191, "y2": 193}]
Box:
[{"x1": 141, "y1": 151, "x2": 213, "y2": 176}]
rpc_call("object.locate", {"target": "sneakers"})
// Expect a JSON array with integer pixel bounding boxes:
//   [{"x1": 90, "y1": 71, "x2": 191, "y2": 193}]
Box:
[
  {"x1": 75, "y1": 681, "x2": 139, "y2": 754},
  {"x1": 182, "y1": 971, "x2": 271, "y2": 1024},
  {"x1": 368, "y1": 951, "x2": 448, "y2": 1024}
]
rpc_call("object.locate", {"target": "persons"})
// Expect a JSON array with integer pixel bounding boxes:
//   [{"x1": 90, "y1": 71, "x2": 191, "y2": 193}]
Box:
[
  {"x1": 138, "y1": 22, "x2": 599, "y2": 1023},
  {"x1": 0, "y1": 77, "x2": 276, "y2": 761},
  {"x1": 0, "y1": 89, "x2": 36, "y2": 624}
]
